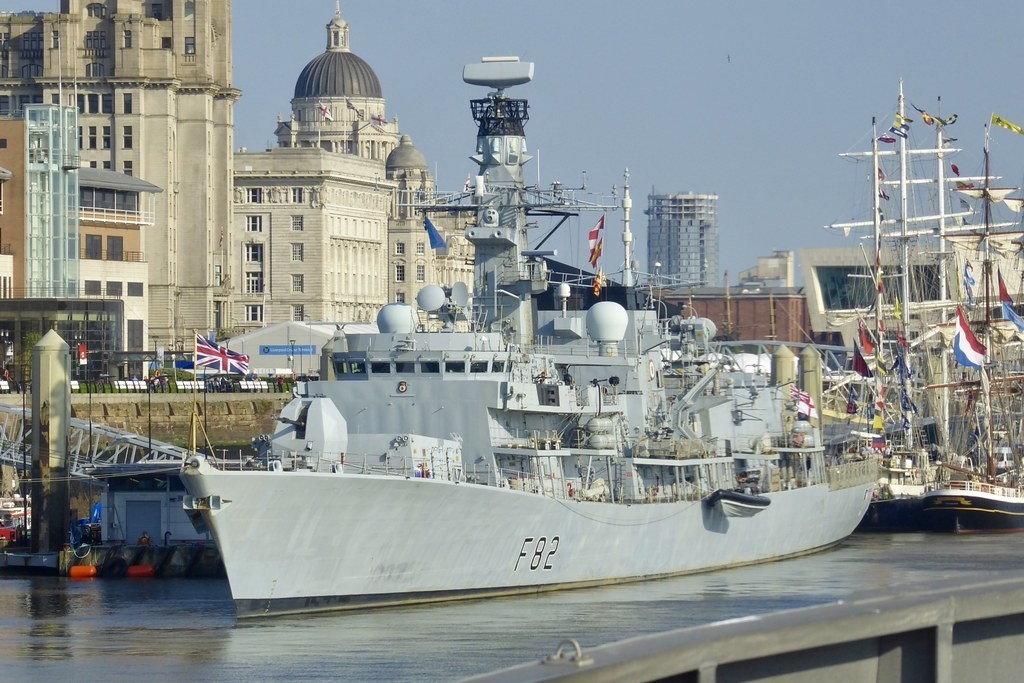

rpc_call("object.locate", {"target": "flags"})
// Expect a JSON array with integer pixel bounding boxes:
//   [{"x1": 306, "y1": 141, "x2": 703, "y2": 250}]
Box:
[
  {"x1": 345, "y1": 100, "x2": 364, "y2": 120},
  {"x1": 195, "y1": 335, "x2": 249, "y2": 375},
  {"x1": 425, "y1": 218, "x2": 446, "y2": 250},
  {"x1": 319, "y1": 102, "x2": 332, "y2": 121},
  {"x1": 463, "y1": 177, "x2": 471, "y2": 192},
  {"x1": 588, "y1": 215, "x2": 606, "y2": 296},
  {"x1": 368, "y1": 115, "x2": 388, "y2": 125},
  {"x1": 789, "y1": 103, "x2": 1024, "y2": 450}
]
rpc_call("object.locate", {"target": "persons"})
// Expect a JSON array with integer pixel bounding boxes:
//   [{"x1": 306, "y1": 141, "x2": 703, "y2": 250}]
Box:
[
  {"x1": 131, "y1": 371, "x2": 312, "y2": 394},
  {"x1": 0, "y1": 365, "x2": 14, "y2": 393}
]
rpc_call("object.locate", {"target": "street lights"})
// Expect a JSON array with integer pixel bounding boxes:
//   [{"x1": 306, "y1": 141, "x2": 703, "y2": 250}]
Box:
[{"x1": 152, "y1": 336, "x2": 159, "y2": 376}]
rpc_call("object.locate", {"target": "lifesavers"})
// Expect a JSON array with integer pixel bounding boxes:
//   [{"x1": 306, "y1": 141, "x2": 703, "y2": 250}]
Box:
[
  {"x1": 101, "y1": 556, "x2": 128, "y2": 577},
  {"x1": 793, "y1": 432, "x2": 806, "y2": 447}
]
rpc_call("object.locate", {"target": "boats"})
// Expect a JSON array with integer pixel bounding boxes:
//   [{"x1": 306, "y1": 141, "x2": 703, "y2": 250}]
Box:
[
  {"x1": 887, "y1": 484, "x2": 926, "y2": 498},
  {"x1": 707, "y1": 487, "x2": 771, "y2": 517},
  {"x1": 174, "y1": 53, "x2": 887, "y2": 621}
]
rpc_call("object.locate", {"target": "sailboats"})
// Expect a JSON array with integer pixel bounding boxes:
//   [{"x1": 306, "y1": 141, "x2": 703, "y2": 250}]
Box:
[{"x1": 817, "y1": 77, "x2": 1024, "y2": 531}]
